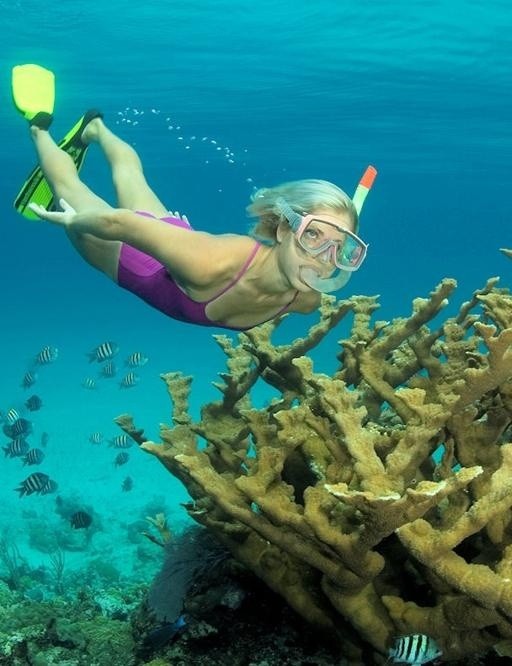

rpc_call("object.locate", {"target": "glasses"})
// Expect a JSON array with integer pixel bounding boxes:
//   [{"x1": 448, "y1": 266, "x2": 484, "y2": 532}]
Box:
[{"x1": 272, "y1": 195, "x2": 369, "y2": 276}]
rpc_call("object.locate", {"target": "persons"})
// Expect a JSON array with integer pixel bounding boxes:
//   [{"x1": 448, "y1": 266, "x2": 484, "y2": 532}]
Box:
[{"x1": 15, "y1": 89, "x2": 362, "y2": 331}]
